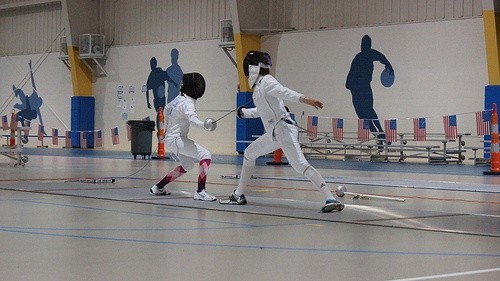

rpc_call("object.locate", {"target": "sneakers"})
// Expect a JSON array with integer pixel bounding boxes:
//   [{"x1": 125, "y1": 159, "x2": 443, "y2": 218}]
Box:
[
  {"x1": 219, "y1": 188, "x2": 247, "y2": 205},
  {"x1": 150, "y1": 184, "x2": 171, "y2": 195},
  {"x1": 321, "y1": 198, "x2": 344, "y2": 213},
  {"x1": 193, "y1": 189, "x2": 217, "y2": 201}
]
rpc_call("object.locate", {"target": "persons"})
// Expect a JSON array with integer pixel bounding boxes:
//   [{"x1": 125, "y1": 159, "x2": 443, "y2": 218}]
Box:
[
  {"x1": 150, "y1": 72, "x2": 217, "y2": 201},
  {"x1": 219, "y1": 51, "x2": 344, "y2": 213}
]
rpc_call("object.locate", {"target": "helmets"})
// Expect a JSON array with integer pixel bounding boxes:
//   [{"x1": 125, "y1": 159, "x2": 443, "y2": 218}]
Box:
[
  {"x1": 179, "y1": 72, "x2": 206, "y2": 100},
  {"x1": 243, "y1": 49, "x2": 272, "y2": 88}
]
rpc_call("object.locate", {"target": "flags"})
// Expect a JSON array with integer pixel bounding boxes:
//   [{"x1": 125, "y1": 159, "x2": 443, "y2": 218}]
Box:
[
  {"x1": 307, "y1": 116, "x2": 318, "y2": 138},
  {"x1": 384, "y1": 120, "x2": 397, "y2": 142},
  {"x1": 65, "y1": 131, "x2": 72, "y2": 147},
  {"x1": 443, "y1": 115, "x2": 457, "y2": 139},
  {"x1": 413, "y1": 117, "x2": 426, "y2": 141},
  {"x1": 24, "y1": 121, "x2": 30, "y2": 136},
  {"x1": 332, "y1": 118, "x2": 343, "y2": 140},
  {"x1": 2, "y1": 116, "x2": 9, "y2": 130},
  {"x1": 127, "y1": 125, "x2": 132, "y2": 141},
  {"x1": 358, "y1": 119, "x2": 369, "y2": 142},
  {"x1": 52, "y1": 128, "x2": 58, "y2": 145},
  {"x1": 38, "y1": 125, "x2": 44, "y2": 140},
  {"x1": 476, "y1": 110, "x2": 490, "y2": 136},
  {"x1": 111, "y1": 127, "x2": 119, "y2": 144},
  {"x1": 79, "y1": 132, "x2": 87, "y2": 149},
  {"x1": 95, "y1": 130, "x2": 102, "y2": 147}
]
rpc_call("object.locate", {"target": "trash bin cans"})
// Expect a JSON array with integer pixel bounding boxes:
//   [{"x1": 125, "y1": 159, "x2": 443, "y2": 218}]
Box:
[{"x1": 128, "y1": 119, "x2": 155, "y2": 159}]
